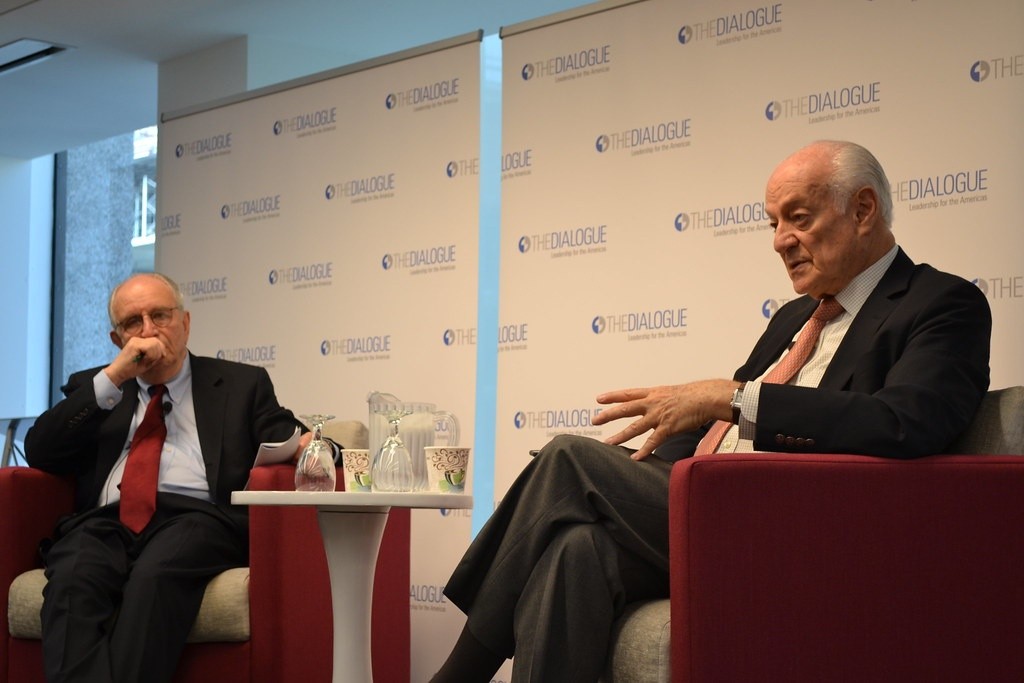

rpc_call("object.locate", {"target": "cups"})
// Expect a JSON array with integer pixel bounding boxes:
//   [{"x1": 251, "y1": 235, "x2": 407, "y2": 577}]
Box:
[
  {"x1": 340, "y1": 449, "x2": 370, "y2": 493},
  {"x1": 424, "y1": 447, "x2": 470, "y2": 493}
]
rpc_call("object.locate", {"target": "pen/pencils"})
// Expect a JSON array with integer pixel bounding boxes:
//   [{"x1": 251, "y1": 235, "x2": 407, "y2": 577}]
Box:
[{"x1": 131, "y1": 352, "x2": 145, "y2": 363}]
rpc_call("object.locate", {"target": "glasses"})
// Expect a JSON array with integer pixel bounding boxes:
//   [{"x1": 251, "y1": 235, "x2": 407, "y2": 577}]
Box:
[{"x1": 115, "y1": 304, "x2": 183, "y2": 334}]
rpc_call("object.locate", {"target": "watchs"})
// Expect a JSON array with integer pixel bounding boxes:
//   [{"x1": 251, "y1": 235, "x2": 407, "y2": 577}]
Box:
[{"x1": 730, "y1": 381, "x2": 747, "y2": 426}]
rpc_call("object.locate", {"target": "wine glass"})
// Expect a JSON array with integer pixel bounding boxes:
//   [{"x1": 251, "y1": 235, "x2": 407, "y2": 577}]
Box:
[
  {"x1": 372, "y1": 410, "x2": 413, "y2": 492},
  {"x1": 294, "y1": 414, "x2": 337, "y2": 491}
]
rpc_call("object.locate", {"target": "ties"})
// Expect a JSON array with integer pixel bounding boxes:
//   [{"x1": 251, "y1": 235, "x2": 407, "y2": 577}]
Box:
[
  {"x1": 119, "y1": 385, "x2": 167, "y2": 534},
  {"x1": 691, "y1": 297, "x2": 845, "y2": 457}
]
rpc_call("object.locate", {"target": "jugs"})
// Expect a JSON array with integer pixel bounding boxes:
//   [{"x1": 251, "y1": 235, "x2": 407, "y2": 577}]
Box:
[{"x1": 367, "y1": 391, "x2": 460, "y2": 492}]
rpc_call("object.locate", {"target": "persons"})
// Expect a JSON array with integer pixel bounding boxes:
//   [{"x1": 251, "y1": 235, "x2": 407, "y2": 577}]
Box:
[
  {"x1": 24, "y1": 271, "x2": 345, "y2": 683},
  {"x1": 427, "y1": 138, "x2": 993, "y2": 683}
]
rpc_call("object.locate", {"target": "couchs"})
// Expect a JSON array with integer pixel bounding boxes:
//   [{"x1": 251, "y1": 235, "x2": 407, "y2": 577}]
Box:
[
  {"x1": 610, "y1": 386, "x2": 1022, "y2": 682},
  {"x1": 0, "y1": 421, "x2": 413, "y2": 683}
]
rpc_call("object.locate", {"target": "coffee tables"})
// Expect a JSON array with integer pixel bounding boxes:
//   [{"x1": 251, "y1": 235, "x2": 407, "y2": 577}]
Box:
[{"x1": 229, "y1": 490, "x2": 475, "y2": 683}]
